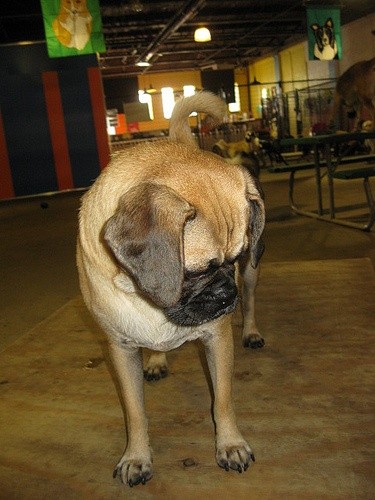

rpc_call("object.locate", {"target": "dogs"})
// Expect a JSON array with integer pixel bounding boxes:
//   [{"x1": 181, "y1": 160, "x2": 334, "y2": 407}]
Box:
[{"x1": 76, "y1": 92, "x2": 266, "y2": 488}]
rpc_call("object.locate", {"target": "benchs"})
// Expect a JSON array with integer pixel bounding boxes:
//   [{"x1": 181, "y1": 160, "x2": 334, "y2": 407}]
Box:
[{"x1": 268, "y1": 155, "x2": 375, "y2": 180}]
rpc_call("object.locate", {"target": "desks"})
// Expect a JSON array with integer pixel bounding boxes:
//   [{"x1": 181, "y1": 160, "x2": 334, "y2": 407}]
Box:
[{"x1": 280, "y1": 132, "x2": 375, "y2": 230}]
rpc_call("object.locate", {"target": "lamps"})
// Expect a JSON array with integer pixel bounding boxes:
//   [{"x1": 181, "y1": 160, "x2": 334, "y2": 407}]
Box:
[{"x1": 194, "y1": 27, "x2": 212, "y2": 42}]
